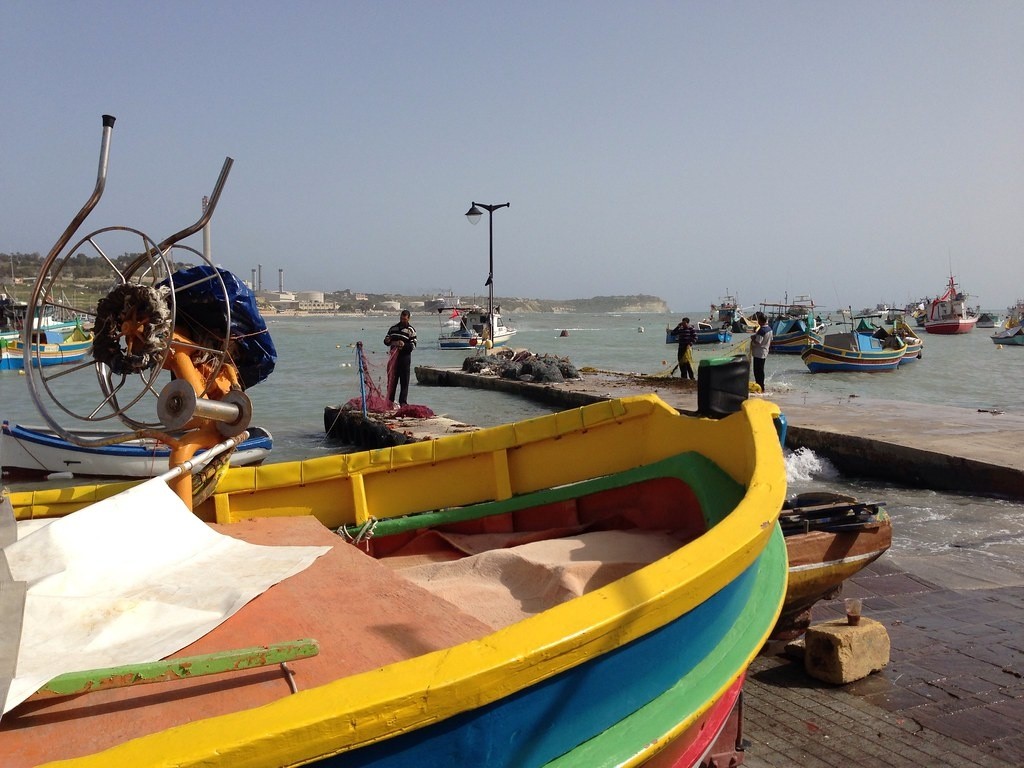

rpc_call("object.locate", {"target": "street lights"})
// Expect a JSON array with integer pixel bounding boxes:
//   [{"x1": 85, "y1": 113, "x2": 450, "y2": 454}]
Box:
[{"x1": 464, "y1": 202, "x2": 511, "y2": 350}]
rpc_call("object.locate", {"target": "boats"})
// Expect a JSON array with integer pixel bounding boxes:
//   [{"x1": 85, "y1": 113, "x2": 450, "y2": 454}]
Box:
[
  {"x1": 436, "y1": 287, "x2": 517, "y2": 351},
  {"x1": 665, "y1": 246, "x2": 1024, "y2": 376},
  {"x1": 0, "y1": 275, "x2": 96, "y2": 373},
  {"x1": 0, "y1": 340, "x2": 891, "y2": 768}
]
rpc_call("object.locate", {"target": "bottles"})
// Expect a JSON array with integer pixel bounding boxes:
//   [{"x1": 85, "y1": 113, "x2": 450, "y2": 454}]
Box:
[{"x1": 44, "y1": 472, "x2": 73, "y2": 479}]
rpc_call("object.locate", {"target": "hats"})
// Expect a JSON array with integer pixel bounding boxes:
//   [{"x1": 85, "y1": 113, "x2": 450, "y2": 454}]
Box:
[{"x1": 756, "y1": 312, "x2": 767, "y2": 322}]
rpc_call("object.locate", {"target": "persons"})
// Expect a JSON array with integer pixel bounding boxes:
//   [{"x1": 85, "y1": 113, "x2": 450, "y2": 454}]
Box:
[
  {"x1": 670, "y1": 317, "x2": 698, "y2": 380},
  {"x1": 383, "y1": 309, "x2": 417, "y2": 406},
  {"x1": 751, "y1": 315, "x2": 772, "y2": 393}
]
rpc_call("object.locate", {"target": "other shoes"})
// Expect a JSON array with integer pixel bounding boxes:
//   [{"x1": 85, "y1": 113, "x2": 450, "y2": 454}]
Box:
[{"x1": 400, "y1": 402, "x2": 408, "y2": 408}]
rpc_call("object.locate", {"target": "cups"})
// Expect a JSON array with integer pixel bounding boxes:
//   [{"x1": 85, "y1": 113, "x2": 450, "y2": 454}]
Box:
[{"x1": 844, "y1": 598, "x2": 862, "y2": 626}]
